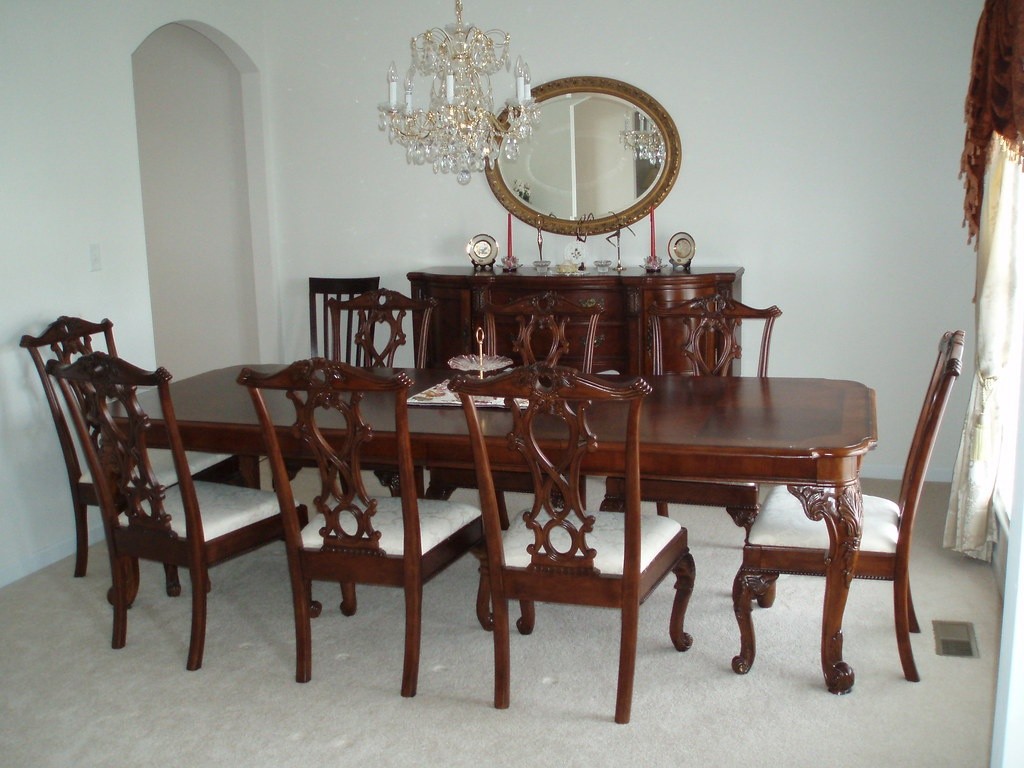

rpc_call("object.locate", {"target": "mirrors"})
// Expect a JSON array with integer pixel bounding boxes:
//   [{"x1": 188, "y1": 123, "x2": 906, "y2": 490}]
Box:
[{"x1": 484, "y1": 76, "x2": 681, "y2": 236}]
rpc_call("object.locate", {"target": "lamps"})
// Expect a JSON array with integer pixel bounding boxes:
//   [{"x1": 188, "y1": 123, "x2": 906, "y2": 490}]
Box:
[
  {"x1": 376, "y1": 0, "x2": 542, "y2": 186},
  {"x1": 617, "y1": 105, "x2": 667, "y2": 165}
]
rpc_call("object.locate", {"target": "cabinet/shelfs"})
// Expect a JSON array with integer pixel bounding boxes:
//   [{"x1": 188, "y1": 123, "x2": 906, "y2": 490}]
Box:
[{"x1": 406, "y1": 266, "x2": 747, "y2": 378}]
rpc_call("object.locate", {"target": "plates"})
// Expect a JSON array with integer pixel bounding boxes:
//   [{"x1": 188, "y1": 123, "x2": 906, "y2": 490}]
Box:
[{"x1": 407, "y1": 378, "x2": 531, "y2": 410}]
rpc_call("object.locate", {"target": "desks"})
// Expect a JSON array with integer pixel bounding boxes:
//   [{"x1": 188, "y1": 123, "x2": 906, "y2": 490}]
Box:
[{"x1": 85, "y1": 364, "x2": 877, "y2": 695}]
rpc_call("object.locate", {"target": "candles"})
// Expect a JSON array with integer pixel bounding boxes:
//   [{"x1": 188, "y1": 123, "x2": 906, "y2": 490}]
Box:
[
  {"x1": 650, "y1": 204, "x2": 655, "y2": 257},
  {"x1": 507, "y1": 210, "x2": 512, "y2": 259}
]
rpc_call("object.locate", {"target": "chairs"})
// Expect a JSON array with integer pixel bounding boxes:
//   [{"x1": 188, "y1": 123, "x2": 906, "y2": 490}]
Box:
[
  {"x1": 273, "y1": 289, "x2": 433, "y2": 498},
  {"x1": 596, "y1": 293, "x2": 784, "y2": 534},
  {"x1": 730, "y1": 329, "x2": 965, "y2": 682},
  {"x1": 19, "y1": 314, "x2": 248, "y2": 596},
  {"x1": 447, "y1": 362, "x2": 695, "y2": 724},
  {"x1": 425, "y1": 293, "x2": 605, "y2": 529},
  {"x1": 237, "y1": 358, "x2": 494, "y2": 698},
  {"x1": 43, "y1": 350, "x2": 322, "y2": 673},
  {"x1": 308, "y1": 275, "x2": 381, "y2": 367}
]
